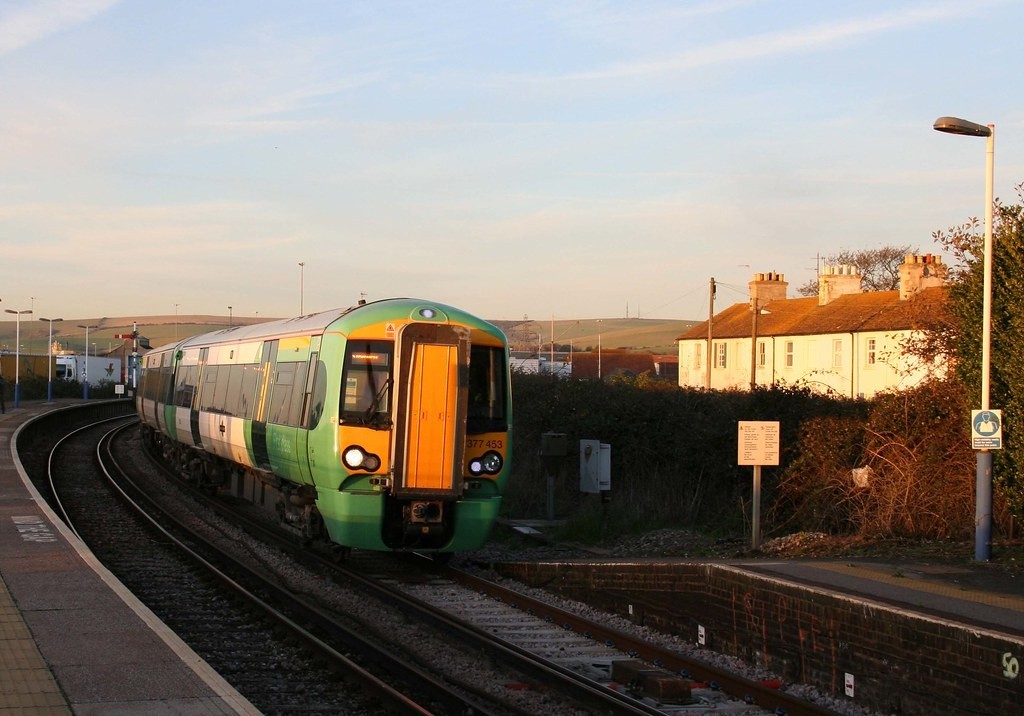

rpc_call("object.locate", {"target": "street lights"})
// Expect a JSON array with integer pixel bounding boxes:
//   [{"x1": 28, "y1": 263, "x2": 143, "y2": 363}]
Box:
[
  {"x1": 931, "y1": 114, "x2": 996, "y2": 562},
  {"x1": 92, "y1": 342, "x2": 97, "y2": 356},
  {"x1": 4, "y1": 309, "x2": 33, "y2": 407},
  {"x1": 40, "y1": 317, "x2": 63, "y2": 402},
  {"x1": 30, "y1": 297, "x2": 37, "y2": 353},
  {"x1": 227, "y1": 306, "x2": 232, "y2": 327},
  {"x1": 77, "y1": 325, "x2": 99, "y2": 401},
  {"x1": 596, "y1": 319, "x2": 602, "y2": 380},
  {"x1": 299, "y1": 262, "x2": 305, "y2": 318},
  {"x1": 751, "y1": 311, "x2": 772, "y2": 393}
]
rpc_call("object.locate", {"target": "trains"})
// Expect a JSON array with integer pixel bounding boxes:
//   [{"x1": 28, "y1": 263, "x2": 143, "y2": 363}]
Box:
[{"x1": 137, "y1": 297, "x2": 513, "y2": 560}]
